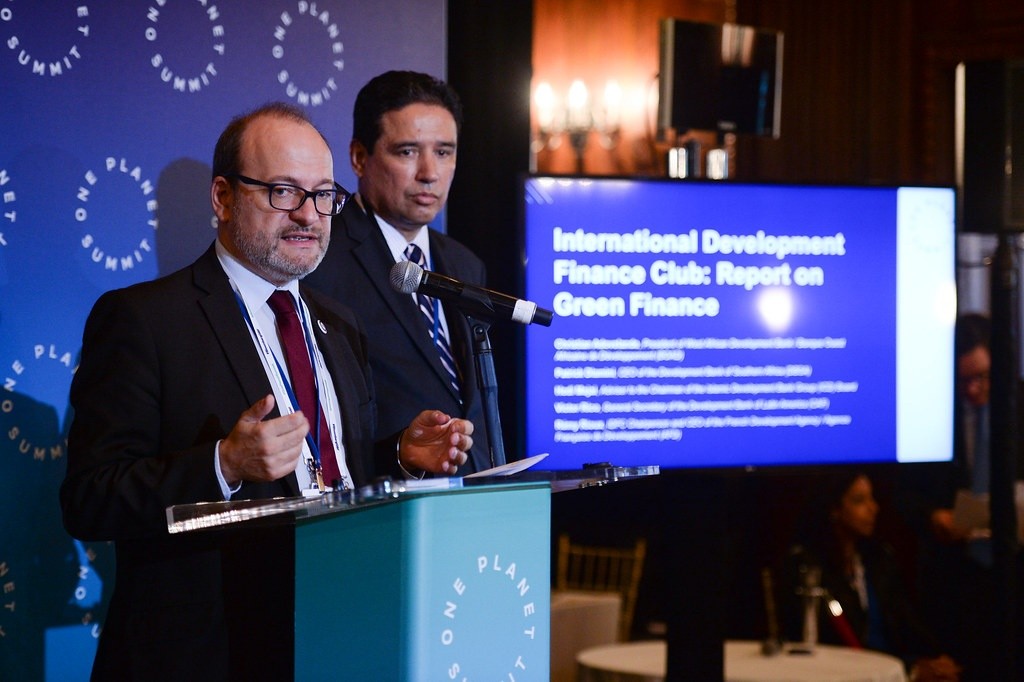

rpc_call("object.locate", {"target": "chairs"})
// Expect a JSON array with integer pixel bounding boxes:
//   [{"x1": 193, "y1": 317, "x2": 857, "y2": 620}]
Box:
[{"x1": 557, "y1": 536, "x2": 647, "y2": 644}]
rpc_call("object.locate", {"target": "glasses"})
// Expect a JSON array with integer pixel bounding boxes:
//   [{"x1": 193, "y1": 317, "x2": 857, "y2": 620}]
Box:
[{"x1": 222, "y1": 171, "x2": 347, "y2": 216}]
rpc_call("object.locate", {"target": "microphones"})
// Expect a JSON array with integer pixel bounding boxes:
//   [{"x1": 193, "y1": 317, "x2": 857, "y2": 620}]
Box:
[{"x1": 389, "y1": 262, "x2": 554, "y2": 327}]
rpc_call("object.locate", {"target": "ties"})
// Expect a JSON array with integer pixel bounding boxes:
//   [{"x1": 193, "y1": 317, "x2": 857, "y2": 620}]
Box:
[
  {"x1": 267, "y1": 289, "x2": 343, "y2": 489},
  {"x1": 404, "y1": 243, "x2": 464, "y2": 406},
  {"x1": 973, "y1": 402, "x2": 992, "y2": 471}
]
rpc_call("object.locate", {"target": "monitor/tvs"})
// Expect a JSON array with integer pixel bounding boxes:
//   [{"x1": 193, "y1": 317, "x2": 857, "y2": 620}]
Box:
[{"x1": 518, "y1": 172, "x2": 961, "y2": 471}]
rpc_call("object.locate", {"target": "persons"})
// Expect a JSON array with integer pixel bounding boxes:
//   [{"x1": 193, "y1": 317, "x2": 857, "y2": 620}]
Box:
[
  {"x1": 776, "y1": 311, "x2": 1024, "y2": 682},
  {"x1": 299, "y1": 68, "x2": 509, "y2": 490},
  {"x1": 55, "y1": 99, "x2": 474, "y2": 682}
]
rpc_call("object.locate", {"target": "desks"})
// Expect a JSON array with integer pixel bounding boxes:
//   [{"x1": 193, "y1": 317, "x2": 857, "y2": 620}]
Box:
[
  {"x1": 574, "y1": 640, "x2": 925, "y2": 682},
  {"x1": 550, "y1": 594, "x2": 621, "y2": 682}
]
